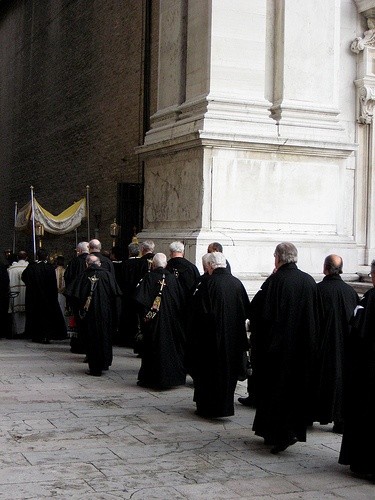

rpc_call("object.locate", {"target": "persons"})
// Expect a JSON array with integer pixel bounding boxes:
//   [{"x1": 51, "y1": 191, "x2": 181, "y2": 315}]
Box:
[
  {"x1": 316, "y1": 255, "x2": 375, "y2": 485},
  {"x1": 238, "y1": 241, "x2": 317, "y2": 455},
  {"x1": 0, "y1": 239, "x2": 257, "y2": 421}
]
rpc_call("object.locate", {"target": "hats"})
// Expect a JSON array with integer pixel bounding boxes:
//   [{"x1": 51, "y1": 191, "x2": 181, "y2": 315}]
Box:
[{"x1": 55, "y1": 256, "x2": 65, "y2": 263}]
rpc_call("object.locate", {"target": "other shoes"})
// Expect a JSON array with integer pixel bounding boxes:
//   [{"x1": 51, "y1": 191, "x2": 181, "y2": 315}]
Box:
[
  {"x1": 101, "y1": 366, "x2": 108, "y2": 371},
  {"x1": 85, "y1": 370, "x2": 100, "y2": 376},
  {"x1": 32, "y1": 337, "x2": 46, "y2": 344},
  {"x1": 270, "y1": 431, "x2": 296, "y2": 454},
  {"x1": 238, "y1": 398, "x2": 256, "y2": 408},
  {"x1": 351, "y1": 472, "x2": 372, "y2": 481}
]
